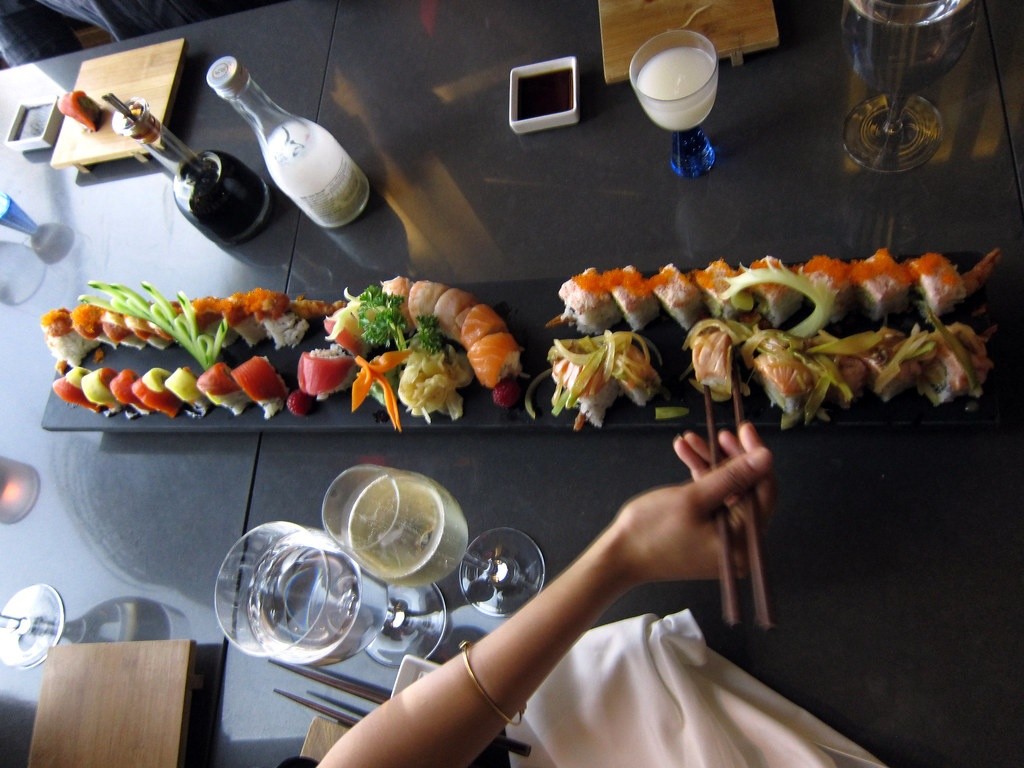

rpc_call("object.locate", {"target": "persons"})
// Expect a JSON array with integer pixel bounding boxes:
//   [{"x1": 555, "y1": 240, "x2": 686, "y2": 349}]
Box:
[
  {"x1": 0, "y1": 0, "x2": 285, "y2": 65},
  {"x1": 315, "y1": 422, "x2": 780, "y2": 768}
]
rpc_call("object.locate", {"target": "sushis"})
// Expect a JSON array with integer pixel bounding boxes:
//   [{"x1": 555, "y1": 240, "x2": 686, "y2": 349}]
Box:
[
  {"x1": 57, "y1": 91, "x2": 102, "y2": 133},
  {"x1": 526, "y1": 249, "x2": 1002, "y2": 429},
  {"x1": 41, "y1": 276, "x2": 530, "y2": 433}
]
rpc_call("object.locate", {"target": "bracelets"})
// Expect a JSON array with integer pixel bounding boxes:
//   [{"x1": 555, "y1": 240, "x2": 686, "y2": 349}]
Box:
[{"x1": 459, "y1": 640, "x2": 526, "y2": 726}]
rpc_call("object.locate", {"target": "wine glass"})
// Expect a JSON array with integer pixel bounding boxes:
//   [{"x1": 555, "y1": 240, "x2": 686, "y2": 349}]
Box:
[
  {"x1": 0, "y1": 583, "x2": 65, "y2": 670},
  {"x1": 320, "y1": 464, "x2": 546, "y2": 619},
  {"x1": 834, "y1": 0, "x2": 983, "y2": 174},
  {"x1": 628, "y1": 30, "x2": 718, "y2": 179},
  {"x1": 214, "y1": 520, "x2": 446, "y2": 667}
]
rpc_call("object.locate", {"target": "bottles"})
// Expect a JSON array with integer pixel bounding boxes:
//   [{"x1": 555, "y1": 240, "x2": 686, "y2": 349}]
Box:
[
  {"x1": 205, "y1": 56, "x2": 370, "y2": 229},
  {"x1": 101, "y1": 92, "x2": 272, "y2": 247}
]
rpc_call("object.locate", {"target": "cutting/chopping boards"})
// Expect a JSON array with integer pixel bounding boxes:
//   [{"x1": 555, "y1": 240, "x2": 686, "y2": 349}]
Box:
[
  {"x1": 598, "y1": 0, "x2": 779, "y2": 85},
  {"x1": 28, "y1": 639, "x2": 197, "y2": 768},
  {"x1": 50, "y1": 36, "x2": 190, "y2": 175}
]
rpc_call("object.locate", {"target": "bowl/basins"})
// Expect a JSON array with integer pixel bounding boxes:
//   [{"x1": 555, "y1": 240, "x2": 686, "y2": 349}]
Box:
[
  {"x1": 5, "y1": 97, "x2": 65, "y2": 151},
  {"x1": 509, "y1": 55, "x2": 579, "y2": 134}
]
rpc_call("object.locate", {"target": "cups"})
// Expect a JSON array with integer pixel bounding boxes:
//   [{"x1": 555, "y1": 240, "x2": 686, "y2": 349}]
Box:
[{"x1": 0, "y1": 456, "x2": 41, "y2": 525}]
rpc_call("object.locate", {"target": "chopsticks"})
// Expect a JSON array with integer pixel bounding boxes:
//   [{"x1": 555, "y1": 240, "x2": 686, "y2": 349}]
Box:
[
  {"x1": 268, "y1": 659, "x2": 532, "y2": 768},
  {"x1": 703, "y1": 355, "x2": 779, "y2": 633}
]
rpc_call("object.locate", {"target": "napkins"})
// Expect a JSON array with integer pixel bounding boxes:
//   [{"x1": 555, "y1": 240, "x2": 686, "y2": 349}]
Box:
[{"x1": 507, "y1": 609, "x2": 887, "y2": 768}]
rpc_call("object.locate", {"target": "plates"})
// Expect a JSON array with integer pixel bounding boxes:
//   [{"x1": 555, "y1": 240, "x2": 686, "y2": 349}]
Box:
[
  {"x1": 41, "y1": 258, "x2": 1024, "y2": 431},
  {"x1": 390, "y1": 654, "x2": 442, "y2": 698}
]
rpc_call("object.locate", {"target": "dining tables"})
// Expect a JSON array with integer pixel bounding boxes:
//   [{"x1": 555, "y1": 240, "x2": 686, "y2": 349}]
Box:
[{"x1": 0, "y1": 0, "x2": 1024, "y2": 768}]
